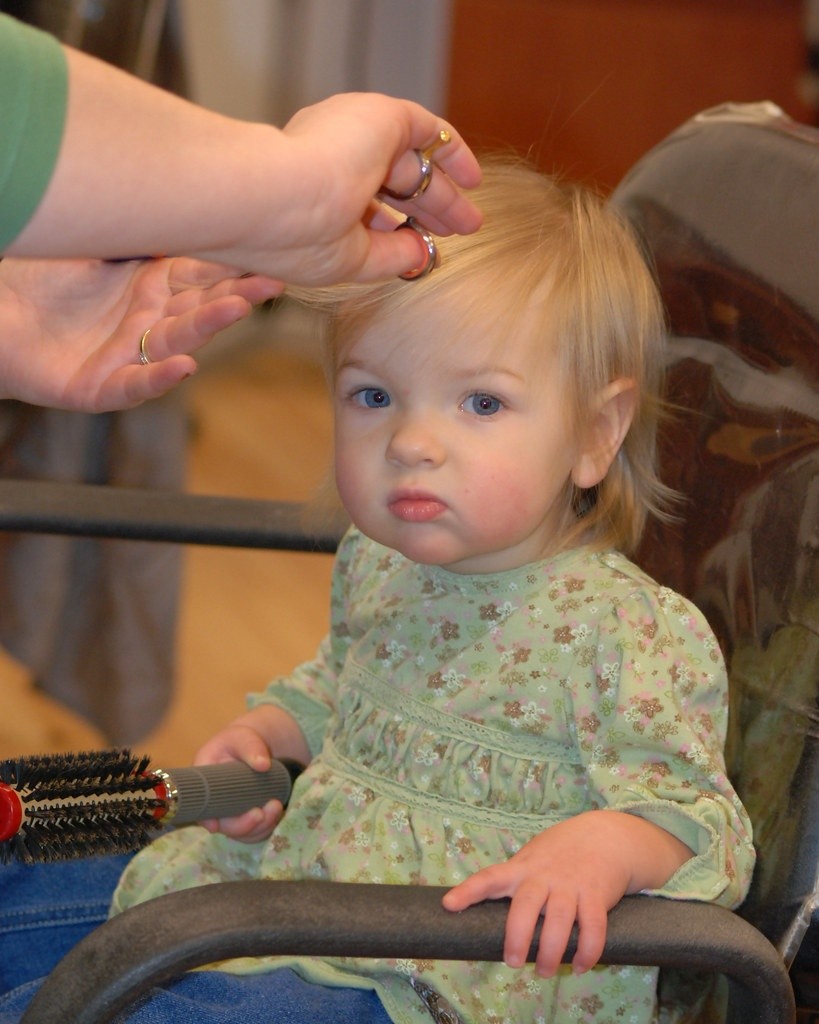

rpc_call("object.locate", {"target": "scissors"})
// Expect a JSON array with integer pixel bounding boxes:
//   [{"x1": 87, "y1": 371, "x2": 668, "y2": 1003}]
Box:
[{"x1": 239, "y1": 147, "x2": 437, "y2": 282}]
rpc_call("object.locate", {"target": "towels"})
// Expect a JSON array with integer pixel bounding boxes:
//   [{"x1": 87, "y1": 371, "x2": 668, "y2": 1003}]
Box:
[{"x1": 0, "y1": 0, "x2": 451, "y2": 755}]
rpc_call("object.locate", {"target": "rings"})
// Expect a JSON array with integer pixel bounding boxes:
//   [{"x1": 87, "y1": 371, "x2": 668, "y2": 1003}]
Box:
[{"x1": 139, "y1": 326, "x2": 153, "y2": 367}]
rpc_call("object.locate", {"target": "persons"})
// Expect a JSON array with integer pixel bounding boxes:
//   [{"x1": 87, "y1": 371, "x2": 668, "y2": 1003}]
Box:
[
  {"x1": 1, "y1": 158, "x2": 757, "y2": 1024},
  {"x1": 0, "y1": 11, "x2": 483, "y2": 412}
]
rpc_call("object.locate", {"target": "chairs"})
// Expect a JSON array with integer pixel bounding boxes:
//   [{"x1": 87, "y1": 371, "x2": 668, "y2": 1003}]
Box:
[{"x1": 0, "y1": 98, "x2": 819, "y2": 1024}]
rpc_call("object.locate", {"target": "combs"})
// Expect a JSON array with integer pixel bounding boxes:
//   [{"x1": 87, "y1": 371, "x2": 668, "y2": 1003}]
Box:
[{"x1": 0, "y1": 747, "x2": 309, "y2": 863}]
